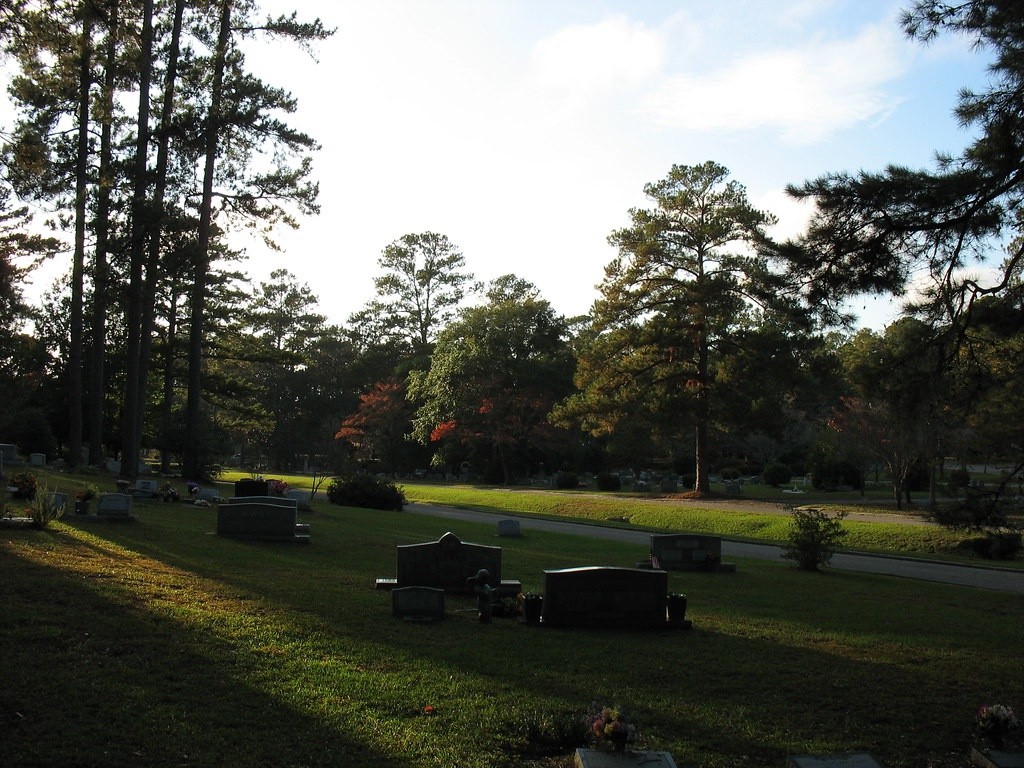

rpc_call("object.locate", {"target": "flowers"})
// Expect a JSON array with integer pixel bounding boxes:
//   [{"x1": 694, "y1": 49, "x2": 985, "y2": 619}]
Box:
[
  {"x1": 75, "y1": 481, "x2": 108, "y2": 503},
  {"x1": 515, "y1": 590, "x2": 545, "y2": 600},
  {"x1": 186, "y1": 481, "x2": 202, "y2": 494},
  {"x1": 667, "y1": 591, "x2": 687, "y2": 600}
]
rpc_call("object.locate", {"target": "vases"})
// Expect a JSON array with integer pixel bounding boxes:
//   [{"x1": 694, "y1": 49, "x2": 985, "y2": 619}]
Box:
[
  {"x1": 524, "y1": 599, "x2": 543, "y2": 622},
  {"x1": 668, "y1": 599, "x2": 687, "y2": 619},
  {"x1": 192, "y1": 493, "x2": 197, "y2": 500},
  {"x1": 75, "y1": 500, "x2": 91, "y2": 515}
]
